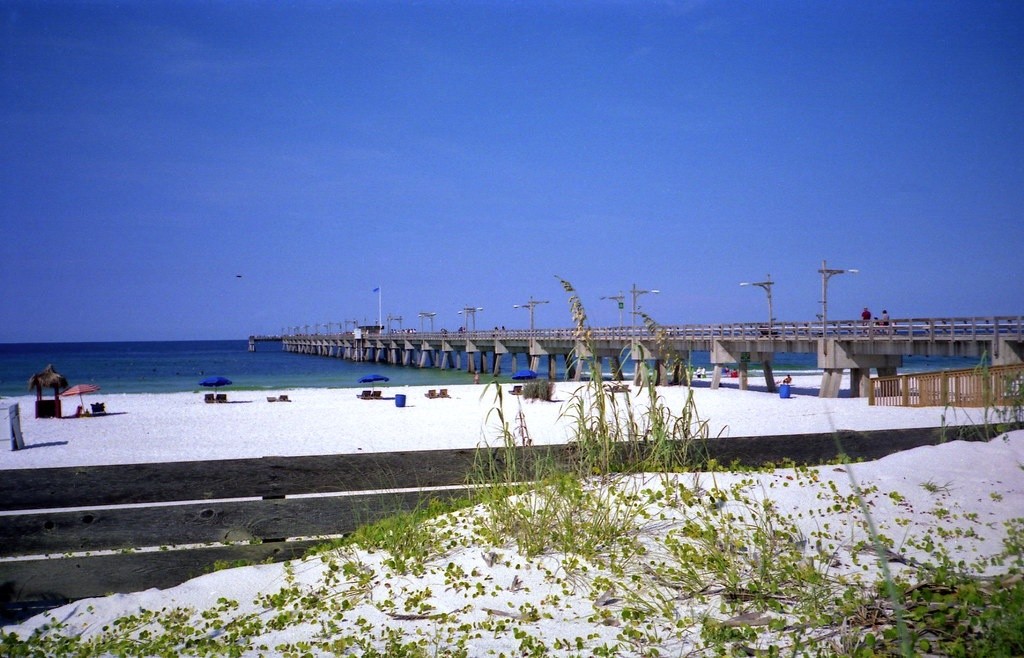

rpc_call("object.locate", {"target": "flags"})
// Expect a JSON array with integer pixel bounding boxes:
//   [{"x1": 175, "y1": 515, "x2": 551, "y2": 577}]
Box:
[{"x1": 374, "y1": 287, "x2": 379, "y2": 292}]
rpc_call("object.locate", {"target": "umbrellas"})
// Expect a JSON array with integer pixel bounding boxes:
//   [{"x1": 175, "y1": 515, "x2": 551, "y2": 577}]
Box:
[
  {"x1": 357, "y1": 374, "x2": 389, "y2": 390},
  {"x1": 512, "y1": 370, "x2": 537, "y2": 384},
  {"x1": 61, "y1": 384, "x2": 101, "y2": 403},
  {"x1": 198, "y1": 376, "x2": 232, "y2": 394}
]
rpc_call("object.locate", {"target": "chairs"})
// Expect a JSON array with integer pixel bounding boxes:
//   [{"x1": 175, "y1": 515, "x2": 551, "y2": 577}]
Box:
[
  {"x1": 267, "y1": 395, "x2": 288, "y2": 402},
  {"x1": 356, "y1": 391, "x2": 381, "y2": 399},
  {"x1": 508, "y1": 386, "x2": 522, "y2": 395},
  {"x1": 424, "y1": 388, "x2": 449, "y2": 399},
  {"x1": 204, "y1": 394, "x2": 227, "y2": 403},
  {"x1": 605, "y1": 385, "x2": 629, "y2": 392},
  {"x1": 91, "y1": 403, "x2": 106, "y2": 415}
]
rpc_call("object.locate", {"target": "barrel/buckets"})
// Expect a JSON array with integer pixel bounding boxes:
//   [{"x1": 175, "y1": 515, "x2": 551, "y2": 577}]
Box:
[
  {"x1": 395, "y1": 394, "x2": 406, "y2": 407},
  {"x1": 779, "y1": 384, "x2": 791, "y2": 398}
]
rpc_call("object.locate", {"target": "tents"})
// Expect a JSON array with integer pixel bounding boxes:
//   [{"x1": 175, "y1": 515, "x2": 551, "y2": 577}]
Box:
[{"x1": 28, "y1": 364, "x2": 68, "y2": 418}]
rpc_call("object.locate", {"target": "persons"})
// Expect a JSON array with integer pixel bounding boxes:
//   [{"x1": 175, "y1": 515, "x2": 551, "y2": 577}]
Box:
[
  {"x1": 882, "y1": 309, "x2": 889, "y2": 333},
  {"x1": 76, "y1": 405, "x2": 83, "y2": 415},
  {"x1": 873, "y1": 317, "x2": 880, "y2": 333},
  {"x1": 458, "y1": 327, "x2": 466, "y2": 336},
  {"x1": 783, "y1": 374, "x2": 791, "y2": 385},
  {"x1": 861, "y1": 307, "x2": 872, "y2": 337},
  {"x1": 731, "y1": 369, "x2": 738, "y2": 377},
  {"x1": 726, "y1": 366, "x2": 729, "y2": 376},
  {"x1": 697, "y1": 366, "x2": 707, "y2": 379},
  {"x1": 441, "y1": 328, "x2": 447, "y2": 337},
  {"x1": 391, "y1": 328, "x2": 417, "y2": 336},
  {"x1": 473, "y1": 371, "x2": 479, "y2": 384},
  {"x1": 493, "y1": 326, "x2": 506, "y2": 337}
]
